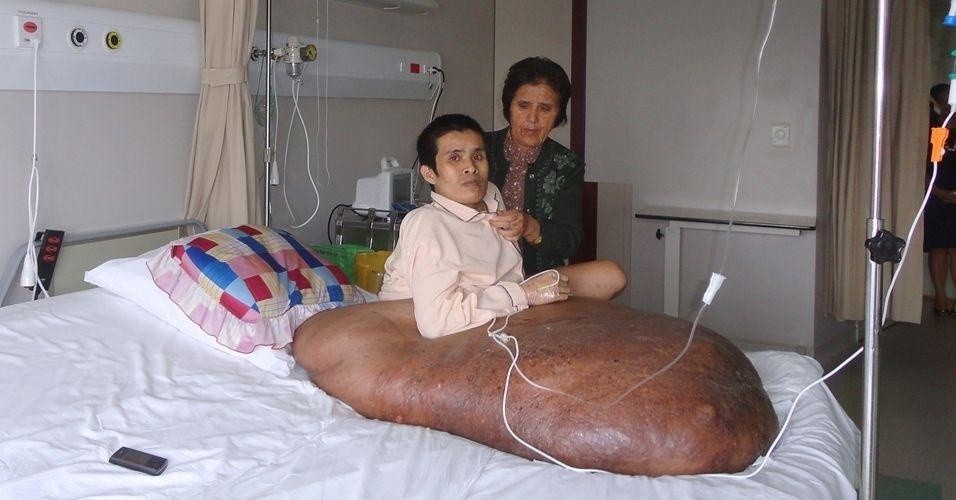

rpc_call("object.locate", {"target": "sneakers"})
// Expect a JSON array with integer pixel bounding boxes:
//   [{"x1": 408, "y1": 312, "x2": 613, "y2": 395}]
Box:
[{"x1": 933, "y1": 302, "x2": 956, "y2": 320}]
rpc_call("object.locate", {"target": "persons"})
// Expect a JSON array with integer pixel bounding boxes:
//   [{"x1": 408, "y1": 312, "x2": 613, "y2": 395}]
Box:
[
  {"x1": 483, "y1": 56, "x2": 584, "y2": 279},
  {"x1": 377, "y1": 114, "x2": 628, "y2": 339},
  {"x1": 923, "y1": 82, "x2": 956, "y2": 319}
]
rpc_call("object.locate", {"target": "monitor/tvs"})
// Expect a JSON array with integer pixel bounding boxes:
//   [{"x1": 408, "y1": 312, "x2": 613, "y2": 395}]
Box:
[{"x1": 356, "y1": 169, "x2": 416, "y2": 219}]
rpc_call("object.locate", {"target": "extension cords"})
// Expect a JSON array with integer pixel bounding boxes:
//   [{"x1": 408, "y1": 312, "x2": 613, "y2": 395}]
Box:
[{"x1": 29, "y1": 229, "x2": 64, "y2": 292}]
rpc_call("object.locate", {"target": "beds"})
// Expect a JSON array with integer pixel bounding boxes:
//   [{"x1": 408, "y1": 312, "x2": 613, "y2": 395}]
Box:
[{"x1": 0, "y1": 254, "x2": 859, "y2": 499}]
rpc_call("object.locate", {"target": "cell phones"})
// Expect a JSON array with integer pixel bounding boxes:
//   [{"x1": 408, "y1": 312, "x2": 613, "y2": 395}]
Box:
[{"x1": 109, "y1": 446, "x2": 168, "y2": 476}]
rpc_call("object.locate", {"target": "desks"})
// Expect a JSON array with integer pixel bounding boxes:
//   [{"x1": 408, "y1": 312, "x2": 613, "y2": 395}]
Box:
[{"x1": 633, "y1": 205, "x2": 815, "y2": 318}]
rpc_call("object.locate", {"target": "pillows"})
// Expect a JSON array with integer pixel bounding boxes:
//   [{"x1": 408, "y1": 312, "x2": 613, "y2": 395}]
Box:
[
  {"x1": 144, "y1": 223, "x2": 366, "y2": 355},
  {"x1": 81, "y1": 223, "x2": 300, "y2": 377}
]
